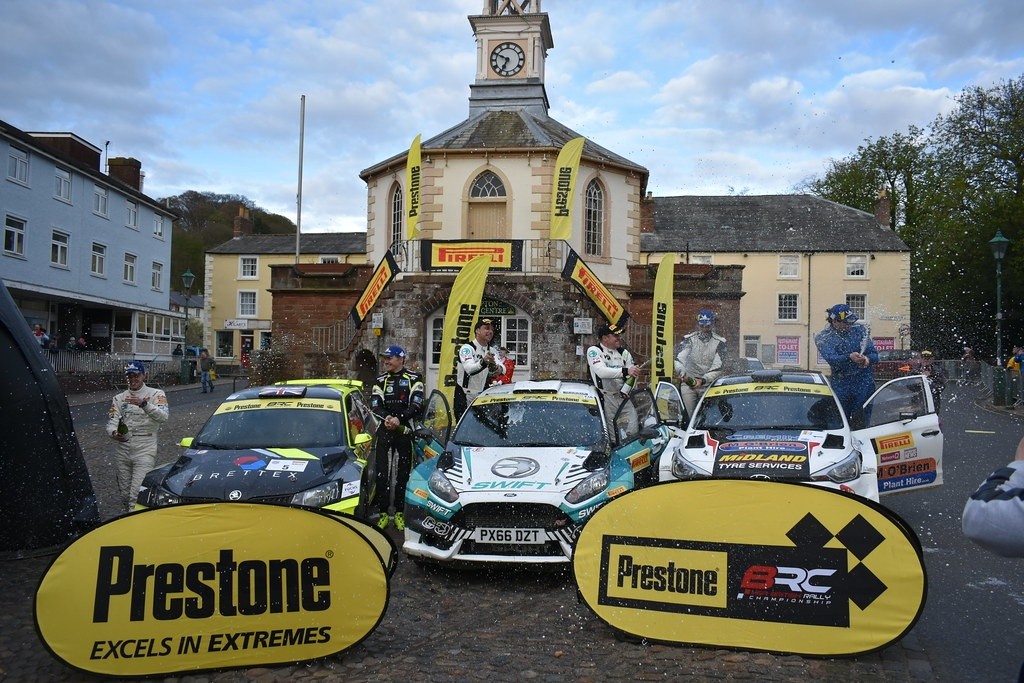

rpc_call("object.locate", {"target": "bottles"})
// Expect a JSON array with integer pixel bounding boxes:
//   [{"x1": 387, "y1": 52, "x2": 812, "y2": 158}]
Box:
[
  {"x1": 118, "y1": 415, "x2": 130, "y2": 443},
  {"x1": 620, "y1": 375, "x2": 635, "y2": 396},
  {"x1": 858, "y1": 359, "x2": 866, "y2": 368},
  {"x1": 682, "y1": 376, "x2": 697, "y2": 386},
  {"x1": 386, "y1": 420, "x2": 413, "y2": 435}
]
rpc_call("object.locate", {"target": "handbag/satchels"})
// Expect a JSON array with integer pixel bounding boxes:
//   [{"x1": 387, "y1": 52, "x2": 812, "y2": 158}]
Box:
[{"x1": 210, "y1": 370, "x2": 215, "y2": 381}]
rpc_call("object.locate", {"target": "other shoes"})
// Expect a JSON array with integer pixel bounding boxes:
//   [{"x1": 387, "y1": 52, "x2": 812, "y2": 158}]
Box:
[
  {"x1": 201, "y1": 391, "x2": 207, "y2": 393},
  {"x1": 210, "y1": 387, "x2": 213, "y2": 392}
]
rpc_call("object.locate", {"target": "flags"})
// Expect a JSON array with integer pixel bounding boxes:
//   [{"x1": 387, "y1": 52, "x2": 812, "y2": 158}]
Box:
[
  {"x1": 550, "y1": 137, "x2": 585, "y2": 240},
  {"x1": 407, "y1": 133, "x2": 422, "y2": 241},
  {"x1": 436, "y1": 253, "x2": 493, "y2": 428},
  {"x1": 651, "y1": 253, "x2": 674, "y2": 398}
]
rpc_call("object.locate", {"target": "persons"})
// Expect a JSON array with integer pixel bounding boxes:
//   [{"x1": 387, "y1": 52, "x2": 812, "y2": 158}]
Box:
[
  {"x1": 31, "y1": 325, "x2": 61, "y2": 373},
  {"x1": 674, "y1": 309, "x2": 728, "y2": 431},
  {"x1": 1007, "y1": 344, "x2": 1024, "y2": 406},
  {"x1": 65, "y1": 337, "x2": 87, "y2": 374},
  {"x1": 815, "y1": 305, "x2": 878, "y2": 431},
  {"x1": 200, "y1": 351, "x2": 216, "y2": 394},
  {"x1": 586, "y1": 322, "x2": 640, "y2": 445},
  {"x1": 906, "y1": 351, "x2": 946, "y2": 416},
  {"x1": 105, "y1": 361, "x2": 169, "y2": 512},
  {"x1": 962, "y1": 435, "x2": 1024, "y2": 683},
  {"x1": 370, "y1": 346, "x2": 425, "y2": 532},
  {"x1": 173, "y1": 344, "x2": 183, "y2": 377},
  {"x1": 453, "y1": 319, "x2": 514, "y2": 425},
  {"x1": 962, "y1": 347, "x2": 974, "y2": 385}
]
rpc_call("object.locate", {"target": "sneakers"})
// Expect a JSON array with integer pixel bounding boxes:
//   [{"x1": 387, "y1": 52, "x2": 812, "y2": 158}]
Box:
[
  {"x1": 375, "y1": 512, "x2": 389, "y2": 529},
  {"x1": 394, "y1": 512, "x2": 409, "y2": 530}
]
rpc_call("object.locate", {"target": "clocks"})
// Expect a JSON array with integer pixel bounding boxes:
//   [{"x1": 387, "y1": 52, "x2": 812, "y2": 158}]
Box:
[{"x1": 491, "y1": 43, "x2": 525, "y2": 76}]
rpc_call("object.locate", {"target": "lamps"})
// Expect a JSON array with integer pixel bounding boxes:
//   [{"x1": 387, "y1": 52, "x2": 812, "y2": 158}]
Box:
[
  {"x1": 425, "y1": 155, "x2": 431, "y2": 162},
  {"x1": 542, "y1": 154, "x2": 547, "y2": 162}
]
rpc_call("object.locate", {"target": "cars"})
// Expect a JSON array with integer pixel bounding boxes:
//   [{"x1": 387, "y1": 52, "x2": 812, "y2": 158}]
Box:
[
  {"x1": 873, "y1": 348, "x2": 921, "y2": 377},
  {"x1": 401, "y1": 380, "x2": 673, "y2": 569},
  {"x1": 134, "y1": 378, "x2": 378, "y2": 517},
  {"x1": 653, "y1": 365, "x2": 944, "y2": 505}
]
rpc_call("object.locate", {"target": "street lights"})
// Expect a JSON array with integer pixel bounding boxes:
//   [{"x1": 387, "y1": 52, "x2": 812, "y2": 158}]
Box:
[
  {"x1": 180, "y1": 265, "x2": 196, "y2": 323},
  {"x1": 988, "y1": 224, "x2": 1012, "y2": 367}
]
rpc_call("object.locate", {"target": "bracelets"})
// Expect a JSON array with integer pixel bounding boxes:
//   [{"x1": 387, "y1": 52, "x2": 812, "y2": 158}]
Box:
[{"x1": 139, "y1": 401, "x2": 147, "y2": 408}]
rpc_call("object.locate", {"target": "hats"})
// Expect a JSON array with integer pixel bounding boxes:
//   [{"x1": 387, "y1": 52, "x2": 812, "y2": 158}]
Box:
[
  {"x1": 598, "y1": 324, "x2": 626, "y2": 338},
  {"x1": 475, "y1": 319, "x2": 497, "y2": 328},
  {"x1": 697, "y1": 310, "x2": 714, "y2": 325},
  {"x1": 124, "y1": 361, "x2": 145, "y2": 375},
  {"x1": 828, "y1": 303, "x2": 858, "y2": 323},
  {"x1": 380, "y1": 346, "x2": 406, "y2": 358},
  {"x1": 921, "y1": 351, "x2": 933, "y2": 358}
]
